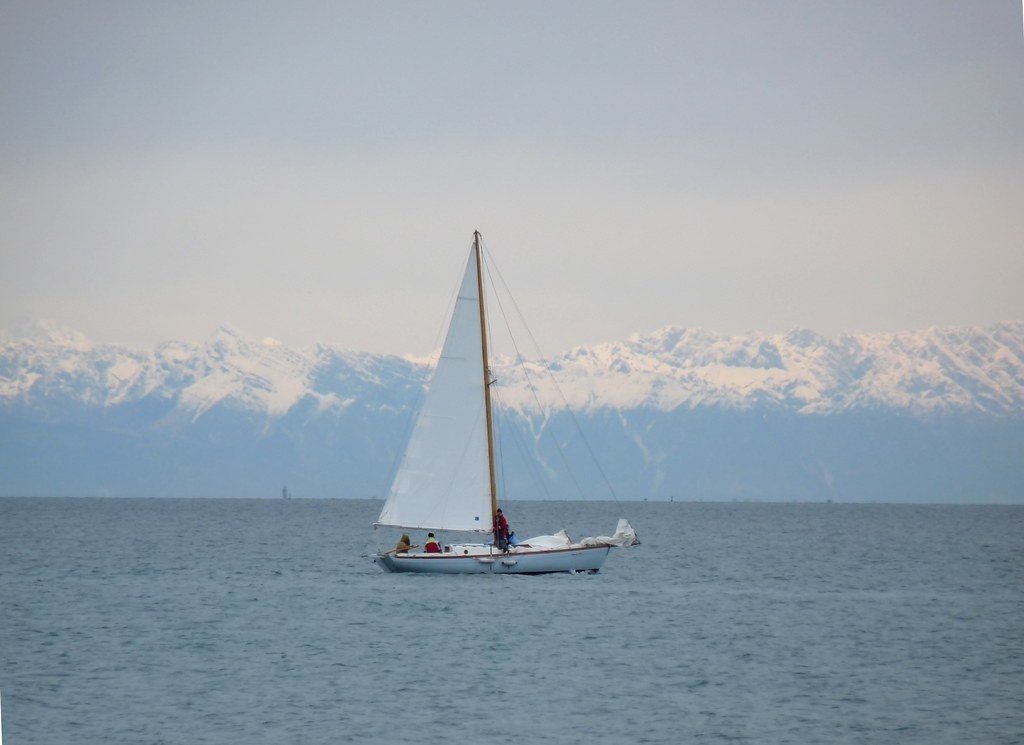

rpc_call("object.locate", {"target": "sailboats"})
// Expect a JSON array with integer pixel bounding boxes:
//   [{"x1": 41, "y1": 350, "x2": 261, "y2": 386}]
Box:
[{"x1": 359, "y1": 223, "x2": 641, "y2": 575}]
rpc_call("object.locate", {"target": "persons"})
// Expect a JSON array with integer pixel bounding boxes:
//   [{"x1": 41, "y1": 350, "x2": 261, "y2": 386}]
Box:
[
  {"x1": 492, "y1": 508, "x2": 514, "y2": 553},
  {"x1": 425, "y1": 532, "x2": 441, "y2": 553},
  {"x1": 396, "y1": 533, "x2": 410, "y2": 553}
]
